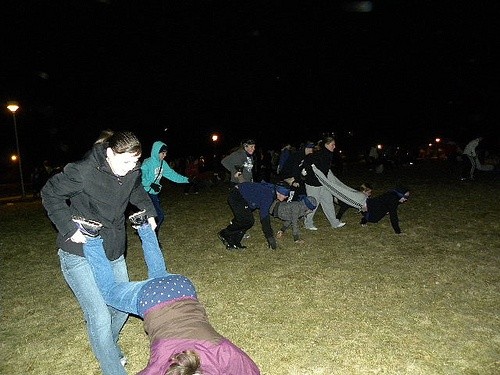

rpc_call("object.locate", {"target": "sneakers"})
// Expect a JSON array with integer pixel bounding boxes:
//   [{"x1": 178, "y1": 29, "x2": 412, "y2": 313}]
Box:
[
  {"x1": 194, "y1": 192, "x2": 198, "y2": 194},
  {"x1": 184, "y1": 193, "x2": 188, "y2": 195},
  {"x1": 95, "y1": 354, "x2": 126, "y2": 366},
  {"x1": 309, "y1": 226, "x2": 317, "y2": 230},
  {"x1": 71, "y1": 214, "x2": 103, "y2": 236},
  {"x1": 129, "y1": 209, "x2": 148, "y2": 226},
  {"x1": 333, "y1": 223, "x2": 346, "y2": 228}
]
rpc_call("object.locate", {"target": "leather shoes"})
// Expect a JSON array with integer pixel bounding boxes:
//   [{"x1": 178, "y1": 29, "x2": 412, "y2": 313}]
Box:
[
  {"x1": 217, "y1": 232, "x2": 232, "y2": 248},
  {"x1": 232, "y1": 240, "x2": 247, "y2": 250}
]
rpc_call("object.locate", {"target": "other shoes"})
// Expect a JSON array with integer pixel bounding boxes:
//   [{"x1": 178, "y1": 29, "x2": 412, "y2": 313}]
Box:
[{"x1": 234, "y1": 164, "x2": 244, "y2": 177}]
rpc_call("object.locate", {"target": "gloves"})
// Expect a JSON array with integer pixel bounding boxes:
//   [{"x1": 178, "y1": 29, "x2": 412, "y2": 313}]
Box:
[
  {"x1": 267, "y1": 236, "x2": 277, "y2": 251},
  {"x1": 188, "y1": 176, "x2": 201, "y2": 184},
  {"x1": 150, "y1": 183, "x2": 161, "y2": 192}
]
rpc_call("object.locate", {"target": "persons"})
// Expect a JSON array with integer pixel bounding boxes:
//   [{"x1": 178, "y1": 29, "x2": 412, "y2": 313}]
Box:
[
  {"x1": 41, "y1": 130, "x2": 159, "y2": 375},
  {"x1": 70, "y1": 209, "x2": 261, "y2": 375},
  {"x1": 134, "y1": 137, "x2": 500, "y2": 250}
]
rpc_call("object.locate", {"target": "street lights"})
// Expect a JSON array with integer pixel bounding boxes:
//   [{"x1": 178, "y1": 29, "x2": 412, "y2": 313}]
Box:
[{"x1": 4, "y1": 100, "x2": 28, "y2": 199}]
